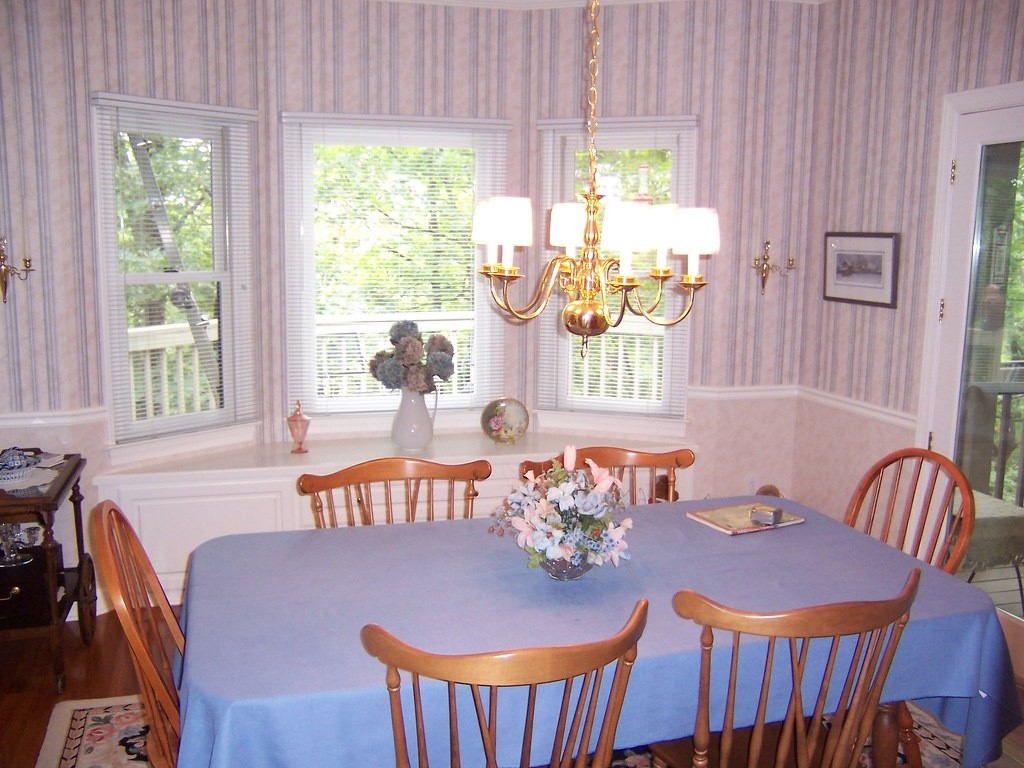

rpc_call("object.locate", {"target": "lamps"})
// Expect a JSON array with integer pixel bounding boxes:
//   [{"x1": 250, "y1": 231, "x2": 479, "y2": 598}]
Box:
[{"x1": 469, "y1": 0, "x2": 720, "y2": 359}]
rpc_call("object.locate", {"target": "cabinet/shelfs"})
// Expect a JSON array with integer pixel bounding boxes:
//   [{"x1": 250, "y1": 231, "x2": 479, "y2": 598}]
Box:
[
  {"x1": 119, "y1": 470, "x2": 688, "y2": 604},
  {"x1": 0, "y1": 451, "x2": 96, "y2": 690}
]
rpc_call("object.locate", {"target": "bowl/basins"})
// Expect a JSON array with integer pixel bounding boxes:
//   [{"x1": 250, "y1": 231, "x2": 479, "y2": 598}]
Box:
[{"x1": 0, "y1": 454, "x2": 41, "y2": 484}]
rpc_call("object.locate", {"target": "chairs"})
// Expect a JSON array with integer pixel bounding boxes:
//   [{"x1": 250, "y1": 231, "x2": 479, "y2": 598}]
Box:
[
  {"x1": 295, "y1": 446, "x2": 694, "y2": 529},
  {"x1": 644, "y1": 569, "x2": 917, "y2": 768},
  {"x1": 834, "y1": 445, "x2": 977, "y2": 768},
  {"x1": 352, "y1": 596, "x2": 651, "y2": 768},
  {"x1": 92, "y1": 500, "x2": 188, "y2": 768}
]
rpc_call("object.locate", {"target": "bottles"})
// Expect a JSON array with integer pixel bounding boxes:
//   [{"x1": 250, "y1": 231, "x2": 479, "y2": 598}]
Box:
[{"x1": 287, "y1": 401, "x2": 311, "y2": 454}]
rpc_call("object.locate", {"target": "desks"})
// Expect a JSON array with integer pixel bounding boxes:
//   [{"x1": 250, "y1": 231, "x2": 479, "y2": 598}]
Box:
[{"x1": 167, "y1": 494, "x2": 1022, "y2": 768}]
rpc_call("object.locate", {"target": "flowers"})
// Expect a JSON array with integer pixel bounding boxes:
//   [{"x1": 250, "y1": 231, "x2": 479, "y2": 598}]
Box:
[
  {"x1": 486, "y1": 454, "x2": 633, "y2": 569},
  {"x1": 367, "y1": 320, "x2": 455, "y2": 396}
]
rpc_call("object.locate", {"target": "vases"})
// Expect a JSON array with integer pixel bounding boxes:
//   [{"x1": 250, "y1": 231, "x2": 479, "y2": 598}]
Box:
[
  {"x1": 388, "y1": 385, "x2": 439, "y2": 450},
  {"x1": 532, "y1": 541, "x2": 594, "y2": 580}
]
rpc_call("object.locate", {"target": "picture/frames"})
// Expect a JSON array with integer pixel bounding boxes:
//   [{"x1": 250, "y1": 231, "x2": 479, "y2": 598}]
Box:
[{"x1": 824, "y1": 231, "x2": 902, "y2": 309}]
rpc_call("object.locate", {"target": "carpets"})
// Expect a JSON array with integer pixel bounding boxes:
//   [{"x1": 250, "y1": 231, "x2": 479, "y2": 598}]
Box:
[{"x1": 34, "y1": 690, "x2": 173, "y2": 768}]
